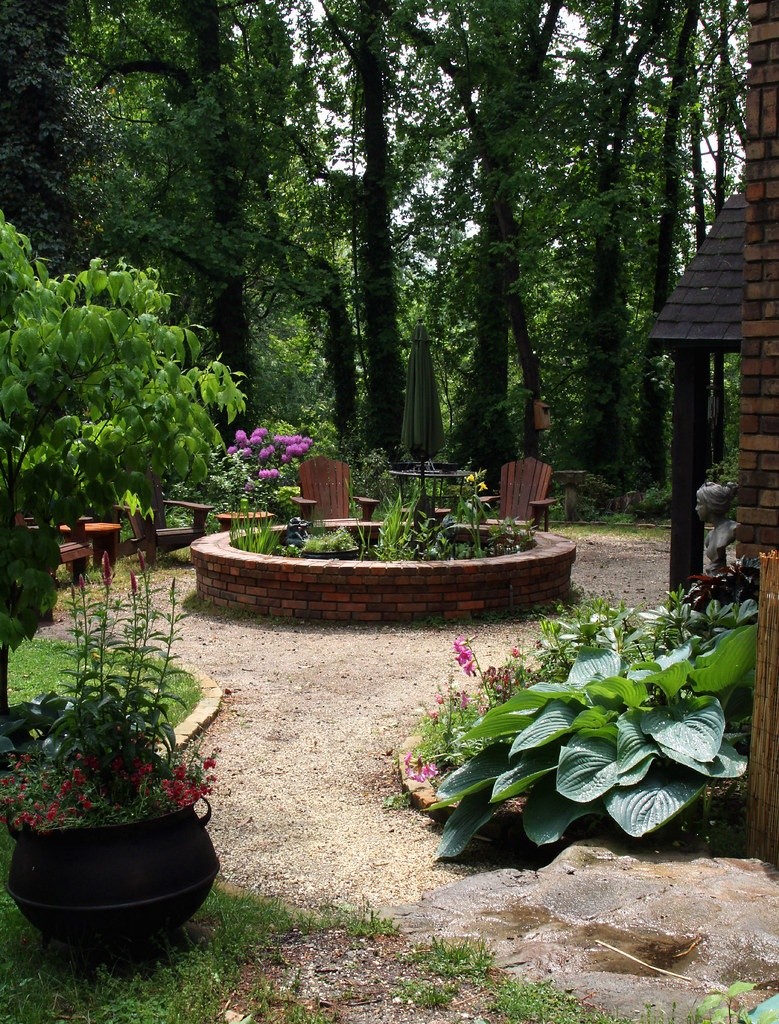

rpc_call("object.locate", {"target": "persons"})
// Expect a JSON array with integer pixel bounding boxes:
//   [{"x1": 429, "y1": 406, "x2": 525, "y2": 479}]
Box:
[{"x1": 695, "y1": 481, "x2": 739, "y2": 575}]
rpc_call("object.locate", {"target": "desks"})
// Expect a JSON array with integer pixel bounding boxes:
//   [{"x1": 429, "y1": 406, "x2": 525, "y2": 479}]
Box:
[
  {"x1": 60, "y1": 523, "x2": 121, "y2": 573},
  {"x1": 215, "y1": 512, "x2": 275, "y2": 532},
  {"x1": 390, "y1": 469, "x2": 470, "y2": 523}
]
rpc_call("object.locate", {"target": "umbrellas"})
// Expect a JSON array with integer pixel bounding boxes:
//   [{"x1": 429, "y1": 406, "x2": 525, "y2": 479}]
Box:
[{"x1": 401, "y1": 318, "x2": 445, "y2": 498}]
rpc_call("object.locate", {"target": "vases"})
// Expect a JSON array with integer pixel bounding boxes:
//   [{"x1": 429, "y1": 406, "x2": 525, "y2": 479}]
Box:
[{"x1": 6, "y1": 795, "x2": 221, "y2": 948}]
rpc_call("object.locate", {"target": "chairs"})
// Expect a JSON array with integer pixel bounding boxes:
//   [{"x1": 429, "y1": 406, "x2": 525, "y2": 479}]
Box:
[
  {"x1": 291, "y1": 456, "x2": 380, "y2": 522},
  {"x1": 468, "y1": 457, "x2": 557, "y2": 532},
  {"x1": 14, "y1": 513, "x2": 92, "y2": 590},
  {"x1": 111, "y1": 466, "x2": 215, "y2": 564}
]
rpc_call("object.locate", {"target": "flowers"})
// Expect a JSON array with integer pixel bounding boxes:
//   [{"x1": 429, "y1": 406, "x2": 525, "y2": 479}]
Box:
[{"x1": 1, "y1": 549, "x2": 216, "y2": 832}]
